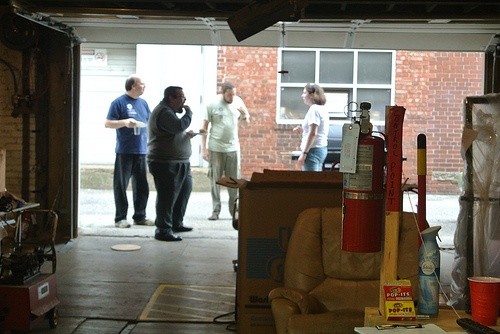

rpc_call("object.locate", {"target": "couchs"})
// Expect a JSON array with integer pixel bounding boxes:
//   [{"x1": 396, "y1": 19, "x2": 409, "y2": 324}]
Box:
[{"x1": 268, "y1": 208, "x2": 430, "y2": 333}]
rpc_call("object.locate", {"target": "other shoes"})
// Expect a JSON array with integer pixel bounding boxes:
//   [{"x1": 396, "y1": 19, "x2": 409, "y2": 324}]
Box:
[{"x1": 208, "y1": 213, "x2": 219, "y2": 220}]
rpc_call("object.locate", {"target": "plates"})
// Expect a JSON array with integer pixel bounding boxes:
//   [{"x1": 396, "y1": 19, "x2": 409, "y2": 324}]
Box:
[{"x1": 189, "y1": 133, "x2": 208, "y2": 135}]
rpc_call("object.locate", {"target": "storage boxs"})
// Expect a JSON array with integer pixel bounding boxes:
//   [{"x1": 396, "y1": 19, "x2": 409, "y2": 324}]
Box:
[{"x1": 216, "y1": 169, "x2": 345, "y2": 334}]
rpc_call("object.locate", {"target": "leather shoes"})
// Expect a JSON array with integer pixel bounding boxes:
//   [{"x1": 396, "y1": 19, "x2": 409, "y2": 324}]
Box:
[
  {"x1": 155, "y1": 229, "x2": 182, "y2": 241},
  {"x1": 173, "y1": 226, "x2": 192, "y2": 232}
]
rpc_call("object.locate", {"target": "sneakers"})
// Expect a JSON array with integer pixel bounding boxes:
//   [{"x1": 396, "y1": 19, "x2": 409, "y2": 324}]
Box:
[
  {"x1": 115, "y1": 219, "x2": 131, "y2": 228},
  {"x1": 133, "y1": 219, "x2": 155, "y2": 226}
]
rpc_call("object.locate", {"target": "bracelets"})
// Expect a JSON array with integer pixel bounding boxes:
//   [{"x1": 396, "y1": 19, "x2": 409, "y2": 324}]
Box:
[
  {"x1": 240, "y1": 116, "x2": 247, "y2": 120},
  {"x1": 302, "y1": 152, "x2": 309, "y2": 155}
]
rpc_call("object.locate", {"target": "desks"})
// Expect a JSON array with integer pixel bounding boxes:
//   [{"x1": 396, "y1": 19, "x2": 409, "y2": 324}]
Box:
[{"x1": 364, "y1": 307, "x2": 500, "y2": 334}]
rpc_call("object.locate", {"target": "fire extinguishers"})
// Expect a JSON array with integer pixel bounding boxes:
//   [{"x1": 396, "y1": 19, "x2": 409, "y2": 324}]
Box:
[{"x1": 339, "y1": 101, "x2": 386, "y2": 253}]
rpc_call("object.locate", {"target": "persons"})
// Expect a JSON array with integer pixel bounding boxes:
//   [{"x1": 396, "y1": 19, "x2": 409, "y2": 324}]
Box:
[
  {"x1": 293, "y1": 83, "x2": 329, "y2": 171},
  {"x1": 147, "y1": 86, "x2": 196, "y2": 241},
  {"x1": 105, "y1": 75, "x2": 152, "y2": 227},
  {"x1": 201, "y1": 82, "x2": 250, "y2": 220}
]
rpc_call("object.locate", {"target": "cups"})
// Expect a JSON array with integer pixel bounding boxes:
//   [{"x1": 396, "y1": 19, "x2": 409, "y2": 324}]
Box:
[
  {"x1": 468, "y1": 277, "x2": 500, "y2": 326},
  {"x1": 134, "y1": 125, "x2": 141, "y2": 135}
]
